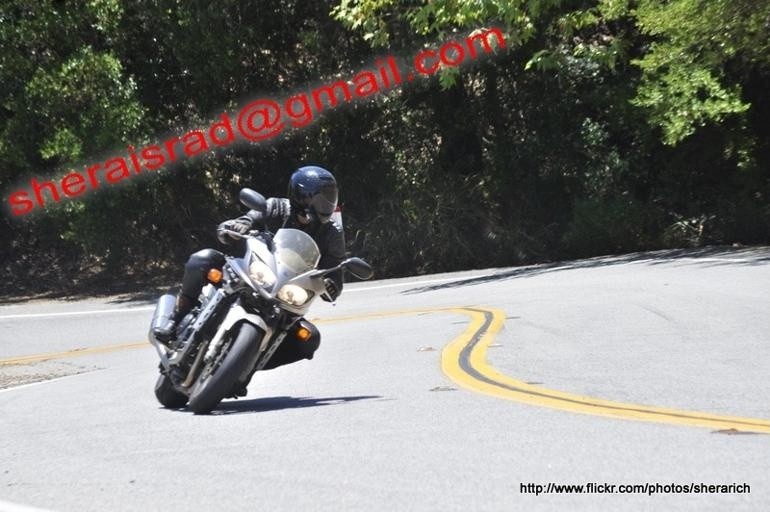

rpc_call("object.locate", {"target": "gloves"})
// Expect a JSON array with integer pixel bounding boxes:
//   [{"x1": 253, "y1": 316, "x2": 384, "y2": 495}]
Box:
[
  {"x1": 224, "y1": 224, "x2": 248, "y2": 243},
  {"x1": 320, "y1": 278, "x2": 339, "y2": 302}
]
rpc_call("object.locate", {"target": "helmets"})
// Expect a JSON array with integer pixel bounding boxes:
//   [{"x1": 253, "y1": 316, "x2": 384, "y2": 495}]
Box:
[{"x1": 289, "y1": 166, "x2": 338, "y2": 225}]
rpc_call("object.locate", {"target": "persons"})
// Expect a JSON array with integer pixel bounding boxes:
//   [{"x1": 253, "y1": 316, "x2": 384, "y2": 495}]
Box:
[{"x1": 152, "y1": 166, "x2": 344, "y2": 395}]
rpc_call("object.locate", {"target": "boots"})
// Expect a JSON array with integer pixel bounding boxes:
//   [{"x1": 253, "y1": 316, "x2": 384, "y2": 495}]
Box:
[{"x1": 153, "y1": 292, "x2": 195, "y2": 340}]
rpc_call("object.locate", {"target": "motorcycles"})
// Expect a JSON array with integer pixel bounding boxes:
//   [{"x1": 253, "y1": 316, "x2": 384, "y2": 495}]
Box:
[{"x1": 148, "y1": 187, "x2": 374, "y2": 414}]
